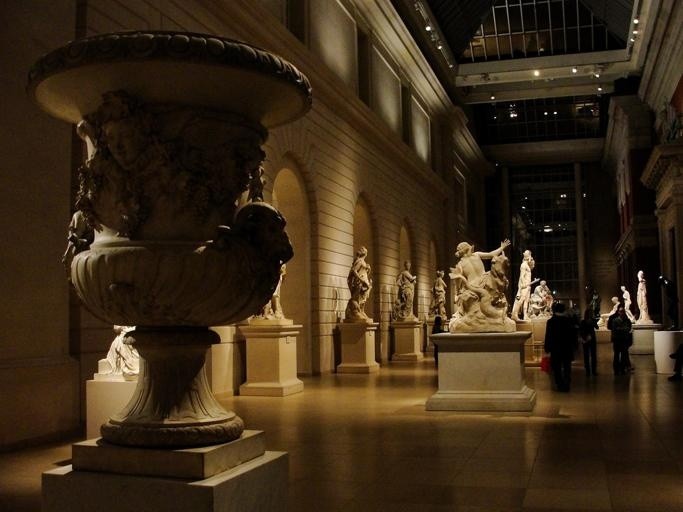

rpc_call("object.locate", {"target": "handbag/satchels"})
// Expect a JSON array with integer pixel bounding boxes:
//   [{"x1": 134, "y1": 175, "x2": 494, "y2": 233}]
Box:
[{"x1": 541, "y1": 356, "x2": 552, "y2": 375}]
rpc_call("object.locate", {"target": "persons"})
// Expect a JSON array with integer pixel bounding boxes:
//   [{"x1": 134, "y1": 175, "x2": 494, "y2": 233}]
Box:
[
  {"x1": 666, "y1": 343, "x2": 682, "y2": 382},
  {"x1": 596, "y1": 296, "x2": 620, "y2": 328},
  {"x1": 430, "y1": 315, "x2": 448, "y2": 366},
  {"x1": 620, "y1": 285, "x2": 633, "y2": 323},
  {"x1": 591, "y1": 287, "x2": 601, "y2": 320},
  {"x1": 343, "y1": 237, "x2": 552, "y2": 322},
  {"x1": 539, "y1": 302, "x2": 580, "y2": 392},
  {"x1": 104, "y1": 324, "x2": 142, "y2": 374},
  {"x1": 578, "y1": 309, "x2": 600, "y2": 380},
  {"x1": 101, "y1": 116, "x2": 146, "y2": 172},
  {"x1": 606, "y1": 301, "x2": 633, "y2": 378},
  {"x1": 636, "y1": 270, "x2": 650, "y2": 319}
]
